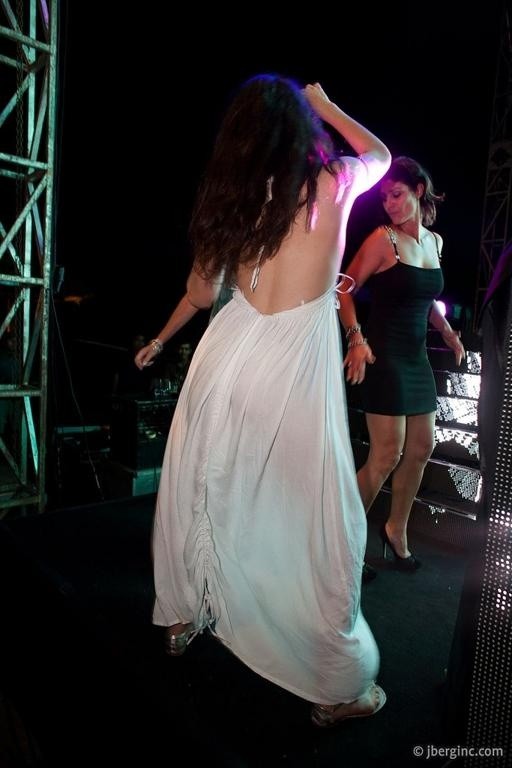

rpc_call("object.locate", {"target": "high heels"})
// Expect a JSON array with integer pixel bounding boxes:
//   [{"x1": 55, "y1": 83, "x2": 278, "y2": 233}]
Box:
[{"x1": 380, "y1": 525, "x2": 422, "y2": 568}]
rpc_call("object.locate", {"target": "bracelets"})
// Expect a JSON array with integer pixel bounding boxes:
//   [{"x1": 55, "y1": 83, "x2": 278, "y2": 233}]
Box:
[
  {"x1": 150, "y1": 339, "x2": 163, "y2": 354},
  {"x1": 347, "y1": 338, "x2": 367, "y2": 349},
  {"x1": 346, "y1": 323, "x2": 361, "y2": 340}
]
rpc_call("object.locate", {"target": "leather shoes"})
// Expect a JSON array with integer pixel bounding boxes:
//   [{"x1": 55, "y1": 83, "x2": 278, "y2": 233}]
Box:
[{"x1": 363, "y1": 562, "x2": 378, "y2": 578}]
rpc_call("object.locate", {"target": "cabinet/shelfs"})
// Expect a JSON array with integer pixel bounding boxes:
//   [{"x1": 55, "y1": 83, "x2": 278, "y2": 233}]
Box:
[{"x1": 348, "y1": 336, "x2": 485, "y2": 521}]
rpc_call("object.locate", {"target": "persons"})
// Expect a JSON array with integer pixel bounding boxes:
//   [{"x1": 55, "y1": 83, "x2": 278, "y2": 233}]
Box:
[
  {"x1": 338, "y1": 157, "x2": 466, "y2": 577},
  {"x1": 135, "y1": 75, "x2": 392, "y2": 726},
  {"x1": 173, "y1": 341, "x2": 193, "y2": 385}
]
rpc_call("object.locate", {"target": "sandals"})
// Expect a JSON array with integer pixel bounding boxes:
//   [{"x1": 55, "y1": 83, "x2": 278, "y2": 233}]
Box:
[
  {"x1": 166, "y1": 611, "x2": 216, "y2": 655},
  {"x1": 312, "y1": 685, "x2": 387, "y2": 726}
]
